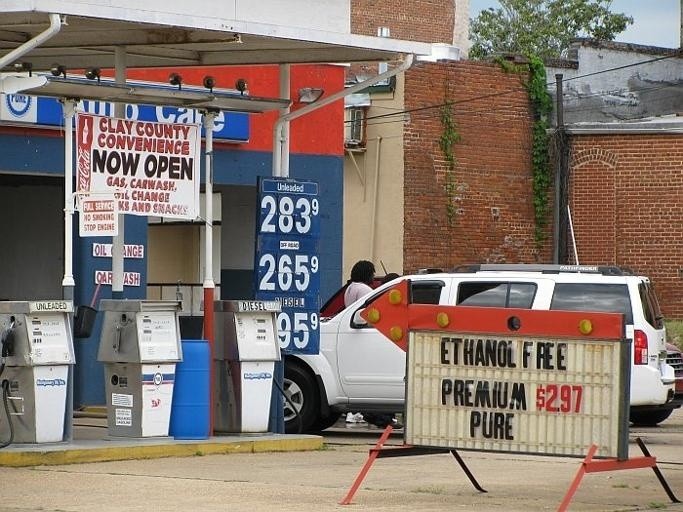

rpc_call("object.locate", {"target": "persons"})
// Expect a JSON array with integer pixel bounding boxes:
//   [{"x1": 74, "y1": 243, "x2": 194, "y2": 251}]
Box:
[{"x1": 345, "y1": 260, "x2": 403, "y2": 430}]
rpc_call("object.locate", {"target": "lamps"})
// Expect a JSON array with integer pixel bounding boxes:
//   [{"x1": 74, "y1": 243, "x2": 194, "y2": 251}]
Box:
[
  {"x1": 50, "y1": 62, "x2": 66, "y2": 79},
  {"x1": 236, "y1": 78, "x2": 248, "y2": 96},
  {"x1": 298, "y1": 88, "x2": 324, "y2": 102},
  {"x1": 203, "y1": 75, "x2": 216, "y2": 93},
  {"x1": 15, "y1": 62, "x2": 32, "y2": 77},
  {"x1": 84, "y1": 67, "x2": 101, "y2": 83},
  {"x1": 169, "y1": 72, "x2": 183, "y2": 91}
]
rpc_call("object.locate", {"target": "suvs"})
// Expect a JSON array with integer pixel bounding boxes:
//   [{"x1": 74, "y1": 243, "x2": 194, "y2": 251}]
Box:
[{"x1": 278, "y1": 255, "x2": 674, "y2": 434}]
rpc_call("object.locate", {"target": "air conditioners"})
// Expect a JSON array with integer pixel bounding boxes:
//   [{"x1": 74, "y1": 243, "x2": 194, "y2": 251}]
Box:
[{"x1": 344, "y1": 107, "x2": 366, "y2": 149}]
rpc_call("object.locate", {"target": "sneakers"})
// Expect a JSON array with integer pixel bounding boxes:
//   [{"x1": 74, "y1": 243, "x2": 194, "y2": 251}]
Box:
[
  {"x1": 368, "y1": 412, "x2": 402, "y2": 429},
  {"x1": 346, "y1": 413, "x2": 367, "y2": 423}
]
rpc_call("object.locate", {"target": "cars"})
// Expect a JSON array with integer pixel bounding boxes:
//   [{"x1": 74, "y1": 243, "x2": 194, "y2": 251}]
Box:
[{"x1": 629, "y1": 337, "x2": 681, "y2": 425}]
